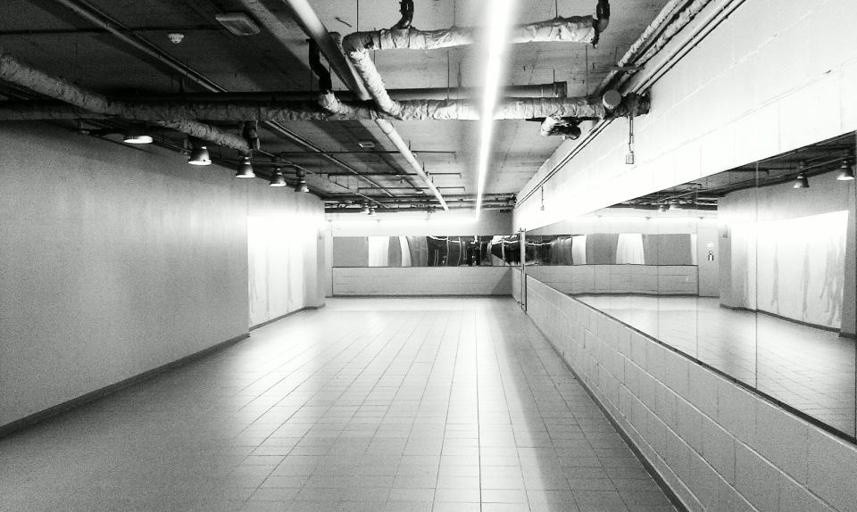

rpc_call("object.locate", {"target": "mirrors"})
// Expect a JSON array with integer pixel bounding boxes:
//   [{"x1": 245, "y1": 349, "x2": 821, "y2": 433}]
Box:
[
  {"x1": 524, "y1": 131, "x2": 856, "y2": 441},
  {"x1": 330, "y1": 234, "x2": 520, "y2": 269},
  {"x1": 524, "y1": 232, "x2": 692, "y2": 269}
]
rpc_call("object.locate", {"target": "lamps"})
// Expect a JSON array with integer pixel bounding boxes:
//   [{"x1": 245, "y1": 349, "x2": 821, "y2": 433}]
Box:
[
  {"x1": 122, "y1": 119, "x2": 310, "y2": 194},
  {"x1": 790, "y1": 157, "x2": 855, "y2": 192},
  {"x1": 360, "y1": 203, "x2": 376, "y2": 215},
  {"x1": 656, "y1": 194, "x2": 683, "y2": 215}
]
